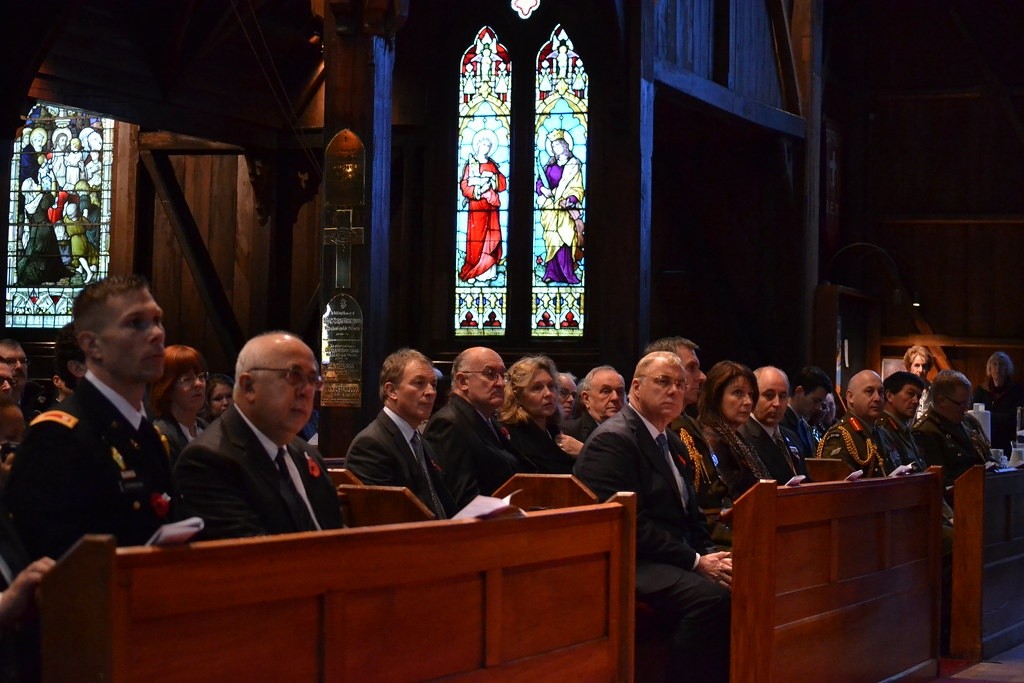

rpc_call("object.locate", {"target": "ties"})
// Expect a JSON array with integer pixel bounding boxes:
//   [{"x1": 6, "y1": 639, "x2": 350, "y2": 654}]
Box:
[
  {"x1": 799, "y1": 420, "x2": 814, "y2": 452},
  {"x1": 904, "y1": 426, "x2": 914, "y2": 447},
  {"x1": 656, "y1": 433, "x2": 671, "y2": 470},
  {"x1": 410, "y1": 431, "x2": 445, "y2": 520},
  {"x1": 772, "y1": 431, "x2": 797, "y2": 479},
  {"x1": 956, "y1": 427, "x2": 970, "y2": 442},
  {"x1": 275, "y1": 448, "x2": 316, "y2": 532},
  {"x1": 137, "y1": 418, "x2": 169, "y2": 492},
  {"x1": 871, "y1": 428, "x2": 886, "y2": 459}
]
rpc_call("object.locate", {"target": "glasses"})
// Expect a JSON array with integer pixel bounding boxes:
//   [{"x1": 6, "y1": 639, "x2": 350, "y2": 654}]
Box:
[
  {"x1": 175, "y1": 370, "x2": 210, "y2": 385},
  {"x1": 936, "y1": 391, "x2": 975, "y2": 410},
  {"x1": 7, "y1": 357, "x2": 30, "y2": 368},
  {"x1": 247, "y1": 365, "x2": 324, "y2": 394},
  {"x1": 457, "y1": 367, "x2": 512, "y2": 383},
  {"x1": 635, "y1": 373, "x2": 690, "y2": 393}
]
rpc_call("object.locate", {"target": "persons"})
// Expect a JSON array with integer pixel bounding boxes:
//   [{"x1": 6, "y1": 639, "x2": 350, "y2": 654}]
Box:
[
  {"x1": 1, "y1": 276, "x2": 344, "y2": 683},
  {"x1": 342, "y1": 337, "x2": 1024, "y2": 683}
]
